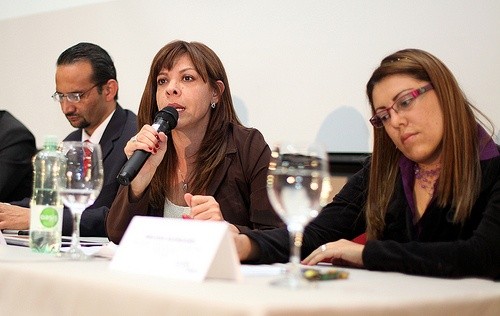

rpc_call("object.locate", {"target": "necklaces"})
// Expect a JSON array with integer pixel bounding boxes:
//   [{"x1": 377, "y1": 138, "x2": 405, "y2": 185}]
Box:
[
  {"x1": 414, "y1": 164, "x2": 440, "y2": 196},
  {"x1": 177, "y1": 167, "x2": 188, "y2": 192}
]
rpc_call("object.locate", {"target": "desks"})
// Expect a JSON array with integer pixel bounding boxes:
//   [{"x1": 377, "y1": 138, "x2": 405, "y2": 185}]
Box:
[{"x1": 0, "y1": 225, "x2": 500, "y2": 316}]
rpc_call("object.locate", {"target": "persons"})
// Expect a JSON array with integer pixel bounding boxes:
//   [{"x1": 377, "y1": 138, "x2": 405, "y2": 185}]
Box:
[
  {"x1": 0, "y1": 43, "x2": 138, "y2": 236},
  {"x1": 300, "y1": 48, "x2": 500, "y2": 283},
  {"x1": 0, "y1": 110, "x2": 36, "y2": 202},
  {"x1": 107, "y1": 41, "x2": 291, "y2": 264}
]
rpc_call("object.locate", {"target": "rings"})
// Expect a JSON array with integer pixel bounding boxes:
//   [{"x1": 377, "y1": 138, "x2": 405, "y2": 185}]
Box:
[
  {"x1": 131, "y1": 137, "x2": 136, "y2": 141},
  {"x1": 322, "y1": 245, "x2": 326, "y2": 250}
]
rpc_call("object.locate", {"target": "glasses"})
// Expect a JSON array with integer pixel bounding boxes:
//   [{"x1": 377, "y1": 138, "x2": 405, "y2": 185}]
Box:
[
  {"x1": 369, "y1": 84, "x2": 434, "y2": 128},
  {"x1": 51, "y1": 83, "x2": 99, "y2": 103}
]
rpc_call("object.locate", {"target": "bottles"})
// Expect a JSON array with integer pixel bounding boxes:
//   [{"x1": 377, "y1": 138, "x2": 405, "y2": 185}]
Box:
[{"x1": 29, "y1": 136, "x2": 67, "y2": 255}]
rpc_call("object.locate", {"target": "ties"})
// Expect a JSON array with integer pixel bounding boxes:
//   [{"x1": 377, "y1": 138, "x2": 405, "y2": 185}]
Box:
[{"x1": 81, "y1": 140, "x2": 93, "y2": 180}]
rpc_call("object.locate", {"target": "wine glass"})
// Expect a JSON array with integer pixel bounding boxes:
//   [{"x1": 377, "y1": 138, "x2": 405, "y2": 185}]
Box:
[
  {"x1": 266, "y1": 141, "x2": 330, "y2": 290},
  {"x1": 57, "y1": 141, "x2": 104, "y2": 260}
]
